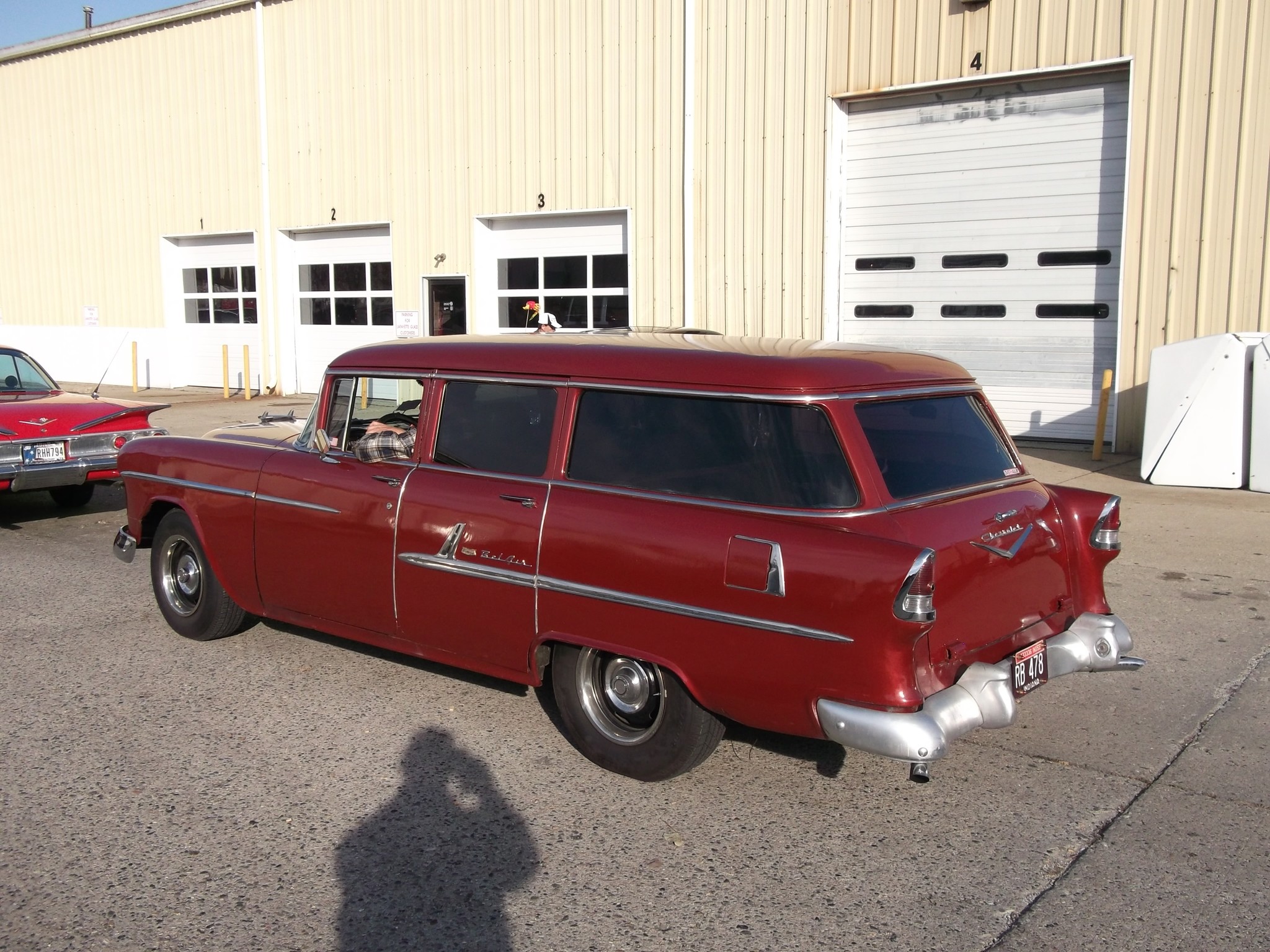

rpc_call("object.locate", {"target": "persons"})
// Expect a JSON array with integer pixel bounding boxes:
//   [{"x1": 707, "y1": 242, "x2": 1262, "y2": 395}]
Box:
[
  {"x1": 534, "y1": 313, "x2": 562, "y2": 333},
  {"x1": 351, "y1": 382, "x2": 476, "y2": 463}
]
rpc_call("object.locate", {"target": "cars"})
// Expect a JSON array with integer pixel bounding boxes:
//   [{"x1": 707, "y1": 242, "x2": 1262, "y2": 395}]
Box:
[
  {"x1": 0, "y1": 347, "x2": 173, "y2": 506},
  {"x1": 113, "y1": 326, "x2": 1148, "y2": 783}
]
